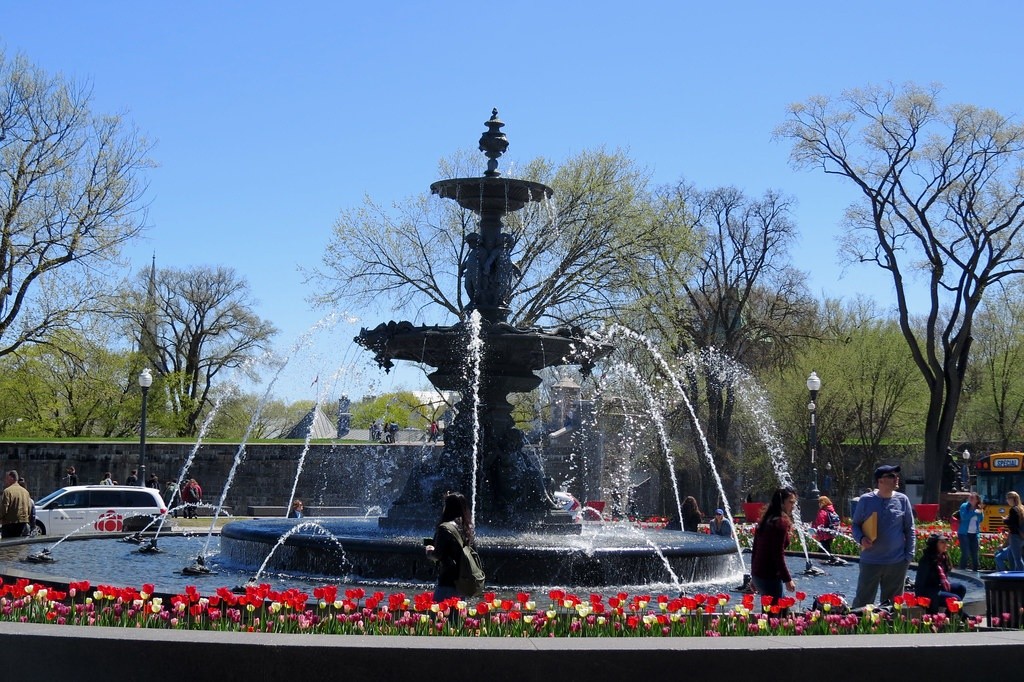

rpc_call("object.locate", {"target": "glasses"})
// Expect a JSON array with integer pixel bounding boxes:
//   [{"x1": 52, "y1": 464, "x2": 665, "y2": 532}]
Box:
[
  {"x1": 716, "y1": 514, "x2": 722, "y2": 515},
  {"x1": 883, "y1": 473, "x2": 901, "y2": 479}
]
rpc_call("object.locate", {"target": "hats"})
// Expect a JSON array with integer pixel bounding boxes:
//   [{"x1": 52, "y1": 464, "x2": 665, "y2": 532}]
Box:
[
  {"x1": 874, "y1": 466, "x2": 900, "y2": 479},
  {"x1": 715, "y1": 509, "x2": 724, "y2": 515},
  {"x1": 927, "y1": 534, "x2": 948, "y2": 542}
]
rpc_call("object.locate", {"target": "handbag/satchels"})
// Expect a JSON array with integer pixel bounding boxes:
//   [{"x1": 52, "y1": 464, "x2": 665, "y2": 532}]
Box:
[
  {"x1": 664, "y1": 519, "x2": 681, "y2": 531},
  {"x1": 950, "y1": 511, "x2": 960, "y2": 531}
]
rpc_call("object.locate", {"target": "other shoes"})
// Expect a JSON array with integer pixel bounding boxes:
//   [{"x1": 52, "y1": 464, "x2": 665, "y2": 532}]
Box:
[{"x1": 968, "y1": 615, "x2": 976, "y2": 620}]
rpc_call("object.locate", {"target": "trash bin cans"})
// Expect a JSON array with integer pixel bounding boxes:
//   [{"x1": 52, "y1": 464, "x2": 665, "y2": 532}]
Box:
[{"x1": 980, "y1": 570, "x2": 1024, "y2": 629}]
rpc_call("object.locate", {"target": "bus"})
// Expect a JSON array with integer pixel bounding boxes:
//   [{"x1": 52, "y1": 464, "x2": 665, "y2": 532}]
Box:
[{"x1": 975, "y1": 450, "x2": 1024, "y2": 533}]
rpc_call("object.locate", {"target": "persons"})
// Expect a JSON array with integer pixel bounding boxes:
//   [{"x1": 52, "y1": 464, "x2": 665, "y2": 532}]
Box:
[
  {"x1": 61, "y1": 465, "x2": 79, "y2": 504},
  {"x1": 383, "y1": 421, "x2": 399, "y2": 443},
  {"x1": 710, "y1": 509, "x2": 733, "y2": 538},
  {"x1": 958, "y1": 491, "x2": 984, "y2": 571},
  {"x1": 993, "y1": 490, "x2": 1023, "y2": 572},
  {"x1": 147, "y1": 473, "x2": 161, "y2": 489},
  {"x1": 809, "y1": 496, "x2": 839, "y2": 552},
  {"x1": 289, "y1": 500, "x2": 304, "y2": 518},
  {"x1": 915, "y1": 533, "x2": 975, "y2": 621},
  {"x1": 101, "y1": 472, "x2": 115, "y2": 485},
  {"x1": 851, "y1": 465, "x2": 916, "y2": 608},
  {"x1": 163, "y1": 478, "x2": 203, "y2": 518},
  {"x1": 125, "y1": 469, "x2": 138, "y2": 486},
  {"x1": 750, "y1": 485, "x2": 797, "y2": 619},
  {"x1": 427, "y1": 420, "x2": 439, "y2": 444},
  {"x1": 1, "y1": 470, "x2": 37, "y2": 539},
  {"x1": 426, "y1": 492, "x2": 477, "y2": 630},
  {"x1": 665, "y1": 496, "x2": 701, "y2": 532}
]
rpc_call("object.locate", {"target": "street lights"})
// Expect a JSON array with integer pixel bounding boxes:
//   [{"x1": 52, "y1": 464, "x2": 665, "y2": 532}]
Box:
[
  {"x1": 962, "y1": 449, "x2": 971, "y2": 492},
  {"x1": 808, "y1": 369, "x2": 832, "y2": 521},
  {"x1": 136, "y1": 367, "x2": 154, "y2": 486}
]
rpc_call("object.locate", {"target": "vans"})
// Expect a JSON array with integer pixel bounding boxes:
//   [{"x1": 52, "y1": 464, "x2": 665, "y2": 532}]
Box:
[{"x1": 27, "y1": 485, "x2": 172, "y2": 535}]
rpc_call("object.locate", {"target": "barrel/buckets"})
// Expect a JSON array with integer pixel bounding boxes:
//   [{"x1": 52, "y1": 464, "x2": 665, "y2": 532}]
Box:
[
  {"x1": 913, "y1": 503, "x2": 940, "y2": 523},
  {"x1": 741, "y1": 502, "x2": 764, "y2": 523},
  {"x1": 587, "y1": 501, "x2": 605, "y2": 520}
]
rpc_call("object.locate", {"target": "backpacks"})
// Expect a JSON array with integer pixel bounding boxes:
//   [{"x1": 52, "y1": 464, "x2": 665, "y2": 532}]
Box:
[
  {"x1": 189, "y1": 485, "x2": 200, "y2": 503},
  {"x1": 443, "y1": 523, "x2": 484, "y2": 597},
  {"x1": 166, "y1": 487, "x2": 175, "y2": 503},
  {"x1": 824, "y1": 509, "x2": 840, "y2": 530}
]
